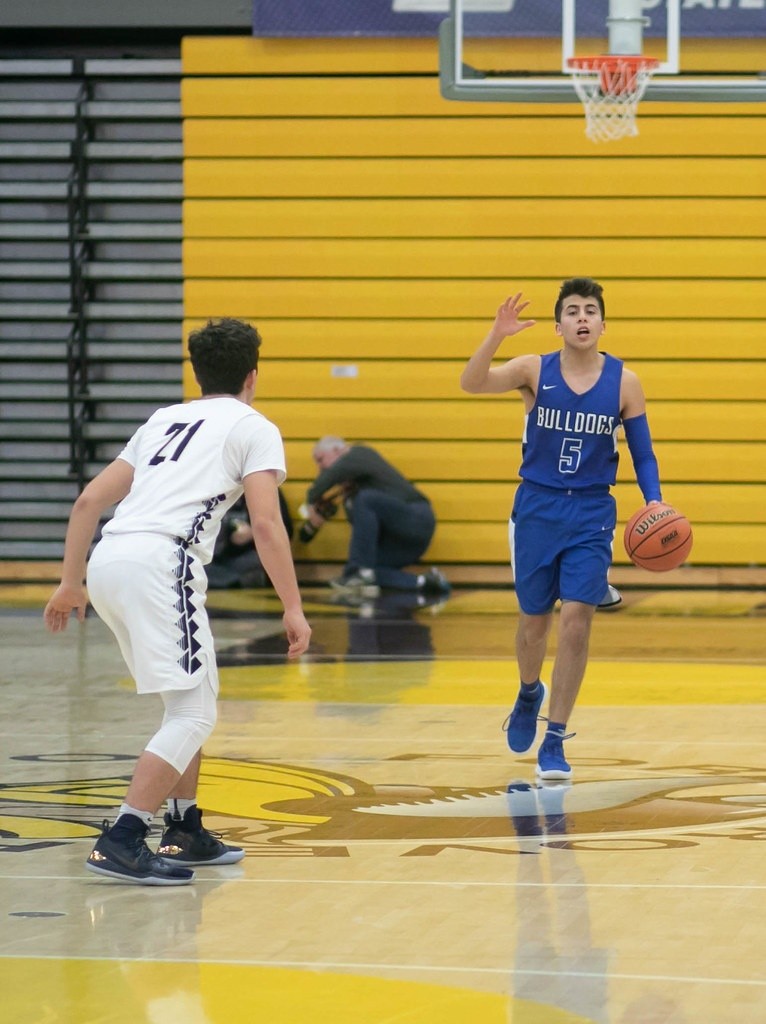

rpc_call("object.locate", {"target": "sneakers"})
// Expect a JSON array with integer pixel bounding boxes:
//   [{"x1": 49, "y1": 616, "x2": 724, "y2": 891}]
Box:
[
  {"x1": 504, "y1": 779, "x2": 571, "y2": 842},
  {"x1": 423, "y1": 567, "x2": 452, "y2": 594},
  {"x1": 83, "y1": 813, "x2": 197, "y2": 887},
  {"x1": 156, "y1": 804, "x2": 246, "y2": 868},
  {"x1": 328, "y1": 570, "x2": 380, "y2": 598},
  {"x1": 535, "y1": 729, "x2": 576, "y2": 780},
  {"x1": 501, "y1": 681, "x2": 549, "y2": 756}
]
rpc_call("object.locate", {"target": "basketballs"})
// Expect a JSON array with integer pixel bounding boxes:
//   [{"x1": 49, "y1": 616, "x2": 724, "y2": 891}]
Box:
[{"x1": 623, "y1": 503, "x2": 694, "y2": 573}]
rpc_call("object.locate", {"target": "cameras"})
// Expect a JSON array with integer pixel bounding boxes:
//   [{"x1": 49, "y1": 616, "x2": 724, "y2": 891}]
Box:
[{"x1": 296, "y1": 501, "x2": 337, "y2": 542}]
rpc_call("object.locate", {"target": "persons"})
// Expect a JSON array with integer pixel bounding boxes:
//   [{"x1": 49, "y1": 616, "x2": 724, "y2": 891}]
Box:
[
  {"x1": 460, "y1": 276, "x2": 677, "y2": 778},
  {"x1": 200, "y1": 434, "x2": 453, "y2": 598},
  {"x1": 41, "y1": 312, "x2": 315, "y2": 887}
]
